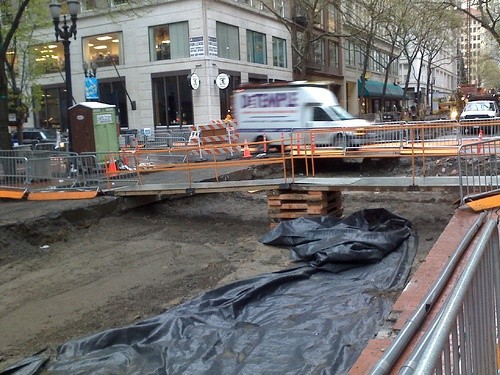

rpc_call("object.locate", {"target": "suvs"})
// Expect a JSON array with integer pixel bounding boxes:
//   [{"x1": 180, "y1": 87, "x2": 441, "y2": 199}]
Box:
[
  {"x1": 13, "y1": 127, "x2": 68, "y2": 147},
  {"x1": 458, "y1": 100, "x2": 499, "y2": 136}
]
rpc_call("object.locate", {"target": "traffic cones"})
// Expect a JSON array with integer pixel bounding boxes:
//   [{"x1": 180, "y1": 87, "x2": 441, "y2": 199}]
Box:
[
  {"x1": 107, "y1": 156, "x2": 119, "y2": 174},
  {"x1": 242, "y1": 139, "x2": 253, "y2": 158}
]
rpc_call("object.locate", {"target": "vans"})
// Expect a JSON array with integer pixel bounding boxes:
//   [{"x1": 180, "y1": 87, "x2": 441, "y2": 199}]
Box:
[{"x1": 232, "y1": 86, "x2": 377, "y2": 151}]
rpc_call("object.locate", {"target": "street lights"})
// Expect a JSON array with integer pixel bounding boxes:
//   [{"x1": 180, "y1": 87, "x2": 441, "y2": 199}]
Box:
[{"x1": 50, "y1": 0, "x2": 80, "y2": 155}]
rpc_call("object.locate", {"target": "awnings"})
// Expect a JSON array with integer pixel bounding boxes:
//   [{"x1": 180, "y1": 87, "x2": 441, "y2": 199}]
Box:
[{"x1": 358, "y1": 79, "x2": 409, "y2": 98}]
